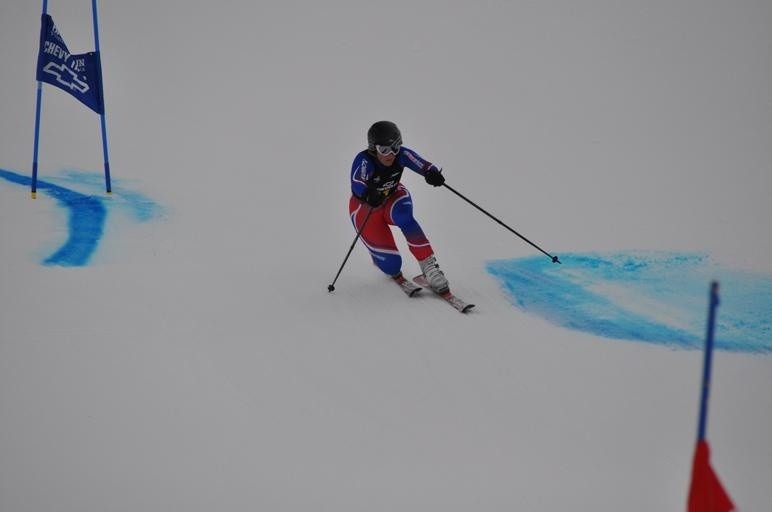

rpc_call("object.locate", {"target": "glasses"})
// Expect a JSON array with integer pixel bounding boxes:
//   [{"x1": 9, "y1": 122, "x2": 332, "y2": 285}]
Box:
[{"x1": 376, "y1": 145, "x2": 400, "y2": 155}]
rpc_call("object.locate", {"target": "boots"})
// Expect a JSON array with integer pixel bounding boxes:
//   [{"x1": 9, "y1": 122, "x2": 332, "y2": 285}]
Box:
[{"x1": 418, "y1": 251, "x2": 452, "y2": 293}]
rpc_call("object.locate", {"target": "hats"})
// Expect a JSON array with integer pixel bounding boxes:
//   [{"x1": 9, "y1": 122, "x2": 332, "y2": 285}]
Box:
[{"x1": 367, "y1": 120, "x2": 404, "y2": 150}]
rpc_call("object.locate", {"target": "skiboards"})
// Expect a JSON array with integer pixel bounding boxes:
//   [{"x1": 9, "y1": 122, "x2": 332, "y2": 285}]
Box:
[{"x1": 391, "y1": 274, "x2": 475, "y2": 313}]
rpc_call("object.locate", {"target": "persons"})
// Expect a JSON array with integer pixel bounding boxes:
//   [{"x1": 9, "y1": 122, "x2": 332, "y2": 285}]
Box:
[{"x1": 347, "y1": 121, "x2": 446, "y2": 297}]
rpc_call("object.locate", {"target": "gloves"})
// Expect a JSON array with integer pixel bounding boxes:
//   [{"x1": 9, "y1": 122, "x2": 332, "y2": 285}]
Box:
[
  {"x1": 361, "y1": 187, "x2": 383, "y2": 207},
  {"x1": 424, "y1": 170, "x2": 446, "y2": 188}
]
